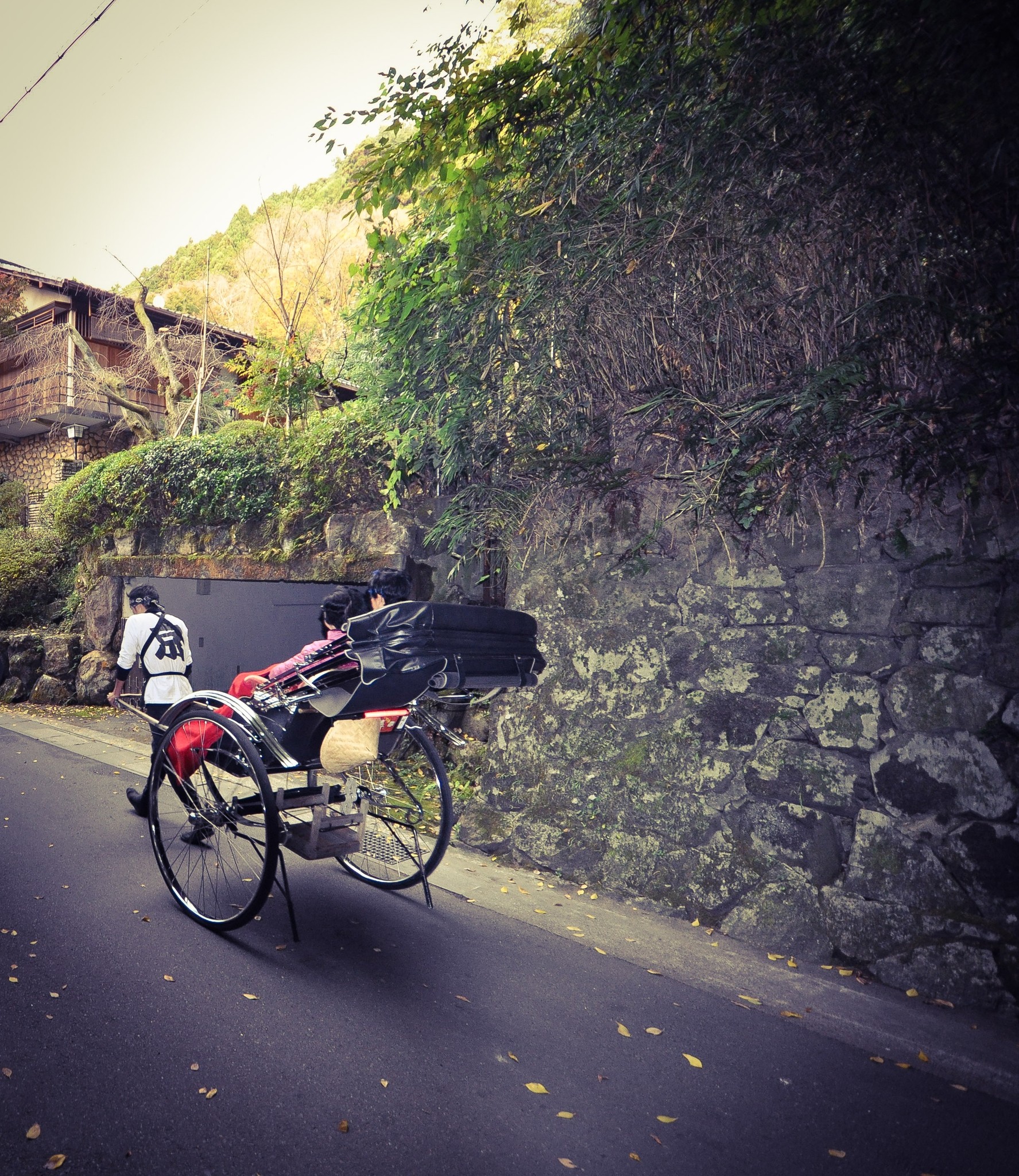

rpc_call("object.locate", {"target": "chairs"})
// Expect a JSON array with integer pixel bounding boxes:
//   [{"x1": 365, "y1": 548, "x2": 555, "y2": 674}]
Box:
[{"x1": 214, "y1": 665, "x2": 363, "y2": 777}]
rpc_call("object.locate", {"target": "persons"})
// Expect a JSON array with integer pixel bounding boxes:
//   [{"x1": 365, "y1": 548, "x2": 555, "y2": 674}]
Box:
[
  {"x1": 104, "y1": 584, "x2": 217, "y2": 846},
  {"x1": 268, "y1": 585, "x2": 372, "y2": 696},
  {"x1": 368, "y1": 567, "x2": 409, "y2": 612}
]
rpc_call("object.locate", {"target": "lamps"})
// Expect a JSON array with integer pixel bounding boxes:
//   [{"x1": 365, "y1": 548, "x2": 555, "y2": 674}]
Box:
[{"x1": 62, "y1": 423, "x2": 89, "y2": 460}]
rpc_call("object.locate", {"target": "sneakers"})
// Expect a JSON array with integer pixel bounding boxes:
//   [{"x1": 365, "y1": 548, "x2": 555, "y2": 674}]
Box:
[
  {"x1": 126, "y1": 788, "x2": 145, "y2": 818},
  {"x1": 180, "y1": 826, "x2": 214, "y2": 842}
]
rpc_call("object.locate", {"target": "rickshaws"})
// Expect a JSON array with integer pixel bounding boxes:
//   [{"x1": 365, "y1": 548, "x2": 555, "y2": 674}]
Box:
[{"x1": 104, "y1": 595, "x2": 546, "y2": 934}]
